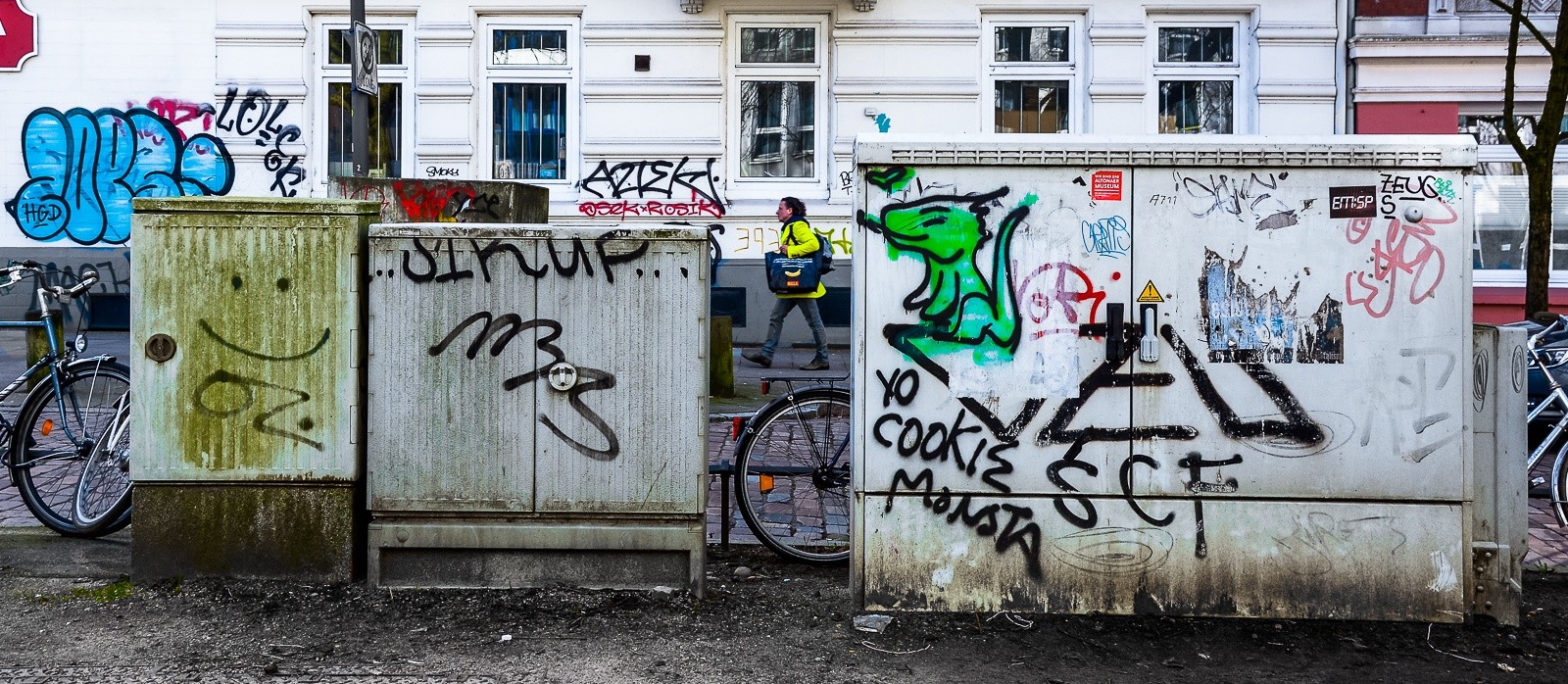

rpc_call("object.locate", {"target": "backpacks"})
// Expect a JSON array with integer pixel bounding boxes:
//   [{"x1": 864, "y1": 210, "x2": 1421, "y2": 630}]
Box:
[{"x1": 790, "y1": 220, "x2": 833, "y2": 274}]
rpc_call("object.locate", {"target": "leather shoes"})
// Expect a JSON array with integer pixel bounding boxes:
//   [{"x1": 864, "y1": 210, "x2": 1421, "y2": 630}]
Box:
[
  {"x1": 799, "y1": 358, "x2": 829, "y2": 370},
  {"x1": 741, "y1": 351, "x2": 771, "y2": 369}
]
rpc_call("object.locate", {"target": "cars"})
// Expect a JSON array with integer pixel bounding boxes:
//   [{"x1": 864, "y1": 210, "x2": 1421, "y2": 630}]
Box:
[{"x1": 1506, "y1": 316, "x2": 1568, "y2": 421}]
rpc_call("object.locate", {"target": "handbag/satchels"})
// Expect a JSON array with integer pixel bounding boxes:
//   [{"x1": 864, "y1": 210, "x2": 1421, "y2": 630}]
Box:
[{"x1": 764, "y1": 250, "x2": 817, "y2": 294}]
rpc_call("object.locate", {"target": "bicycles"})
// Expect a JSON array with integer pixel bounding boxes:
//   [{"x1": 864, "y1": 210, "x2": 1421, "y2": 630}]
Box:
[
  {"x1": 731, "y1": 372, "x2": 852, "y2": 566},
  {"x1": 1527, "y1": 318, "x2": 1568, "y2": 542},
  {"x1": 0, "y1": 260, "x2": 137, "y2": 540}
]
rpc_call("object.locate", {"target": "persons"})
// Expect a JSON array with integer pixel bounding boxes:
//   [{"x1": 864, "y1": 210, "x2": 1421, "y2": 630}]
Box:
[{"x1": 741, "y1": 196, "x2": 830, "y2": 370}]
